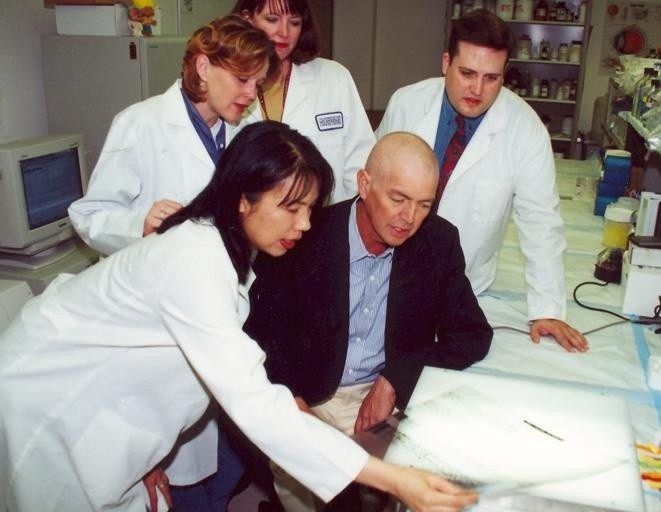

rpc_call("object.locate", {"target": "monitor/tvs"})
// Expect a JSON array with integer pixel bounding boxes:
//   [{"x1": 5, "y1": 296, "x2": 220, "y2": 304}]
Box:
[{"x1": 0, "y1": 133, "x2": 88, "y2": 271}]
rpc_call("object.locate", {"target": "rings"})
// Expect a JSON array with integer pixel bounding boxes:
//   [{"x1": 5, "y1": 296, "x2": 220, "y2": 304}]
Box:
[
  {"x1": 157, "y1": 479, "x2": 168, "y2": 489},
  {"x1": 158, "y1": 211, "x2": 166, "y2": 219}
]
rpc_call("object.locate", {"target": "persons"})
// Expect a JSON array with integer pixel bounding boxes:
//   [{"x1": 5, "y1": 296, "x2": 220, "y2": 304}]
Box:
[
  {"x1": 0, "y1": 119, "x2": 480, "y2": 512},
  {"x1": 223, "y1": 0, "x2": 380, "y2": 208},
  {"x1": 66, "y1": 12, "x2": 282, "y2": 512},
  {"x1": 230, "y1": 131, "x2": 493, "y2": 512},
  {"x1": 368, "y1": 8, "x2": 591, "y2": 355}
]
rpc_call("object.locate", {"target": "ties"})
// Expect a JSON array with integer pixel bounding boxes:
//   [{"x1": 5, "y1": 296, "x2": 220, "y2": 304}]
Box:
[{"x1": 429, "y1": 115, "x2": 466, "y2": 216}]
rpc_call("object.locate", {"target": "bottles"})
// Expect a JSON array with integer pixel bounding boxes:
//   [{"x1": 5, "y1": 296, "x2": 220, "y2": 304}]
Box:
[
  {"x1": 452, "y1": 0, "x2": 586, "y2": 159},
  {"x1": 631, "y1": 68, "x2": 661, "y2": 127},
  {"x1": 602, "y1": 207, "x2": 637, "y2": 249}
]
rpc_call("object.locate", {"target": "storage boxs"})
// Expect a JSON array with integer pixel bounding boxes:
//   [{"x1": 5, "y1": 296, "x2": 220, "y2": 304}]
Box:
[{"x1": 54, "y1": 3, "x2": 162, "y2": 38}]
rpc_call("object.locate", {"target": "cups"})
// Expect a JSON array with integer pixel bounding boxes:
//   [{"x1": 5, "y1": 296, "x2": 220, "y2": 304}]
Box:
[{"x1": 575, "y1": 175, "x2": 597, "y2": 201}]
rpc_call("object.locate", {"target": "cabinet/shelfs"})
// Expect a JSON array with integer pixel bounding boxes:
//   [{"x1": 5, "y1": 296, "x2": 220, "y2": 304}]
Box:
[{"x1": 445, "y1": 0, "x2": 593, "y2": 160}]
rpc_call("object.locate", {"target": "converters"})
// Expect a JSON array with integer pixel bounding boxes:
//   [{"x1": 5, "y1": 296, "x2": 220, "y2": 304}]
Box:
[{"x1": 595, "y1": 249, "x2": 624, "y2": 284}]
